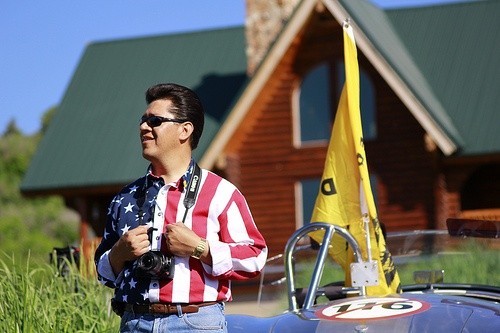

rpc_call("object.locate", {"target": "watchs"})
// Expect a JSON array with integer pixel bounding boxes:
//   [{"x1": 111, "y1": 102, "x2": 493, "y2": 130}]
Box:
[{"x1": 190, "y1": 238, "x2": 206, "y2": 260}]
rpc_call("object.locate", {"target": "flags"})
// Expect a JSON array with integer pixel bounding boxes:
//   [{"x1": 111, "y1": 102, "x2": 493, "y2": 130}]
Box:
[{"x1": 307, "y1": 21, "x2": 404, "y2": 300}]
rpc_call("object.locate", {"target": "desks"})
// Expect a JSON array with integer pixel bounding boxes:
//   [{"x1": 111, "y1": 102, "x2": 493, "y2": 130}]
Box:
[{"x1": 225, "y1": 243, "x2": 312, "y2": 304}]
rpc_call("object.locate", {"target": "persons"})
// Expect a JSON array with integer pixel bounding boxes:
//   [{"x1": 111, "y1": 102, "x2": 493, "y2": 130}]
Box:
[{"x1": 94, "y1": 82, "x2": 269, "y2": 333}]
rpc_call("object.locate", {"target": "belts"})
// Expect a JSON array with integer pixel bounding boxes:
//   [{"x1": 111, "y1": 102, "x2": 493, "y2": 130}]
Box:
[{"x1": 121, "y1": 301, "x2": 217, "y2": 314}]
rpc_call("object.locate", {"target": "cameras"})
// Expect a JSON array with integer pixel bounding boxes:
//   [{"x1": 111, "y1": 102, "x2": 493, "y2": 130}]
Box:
[{"x1": 136, "y1": 251, "x2": 175, "y2": 280}]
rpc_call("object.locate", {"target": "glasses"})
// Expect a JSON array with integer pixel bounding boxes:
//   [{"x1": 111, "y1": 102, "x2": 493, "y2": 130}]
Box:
[{"x1": 139, "y1": 116, "x2": 192, "y2": 127}]
